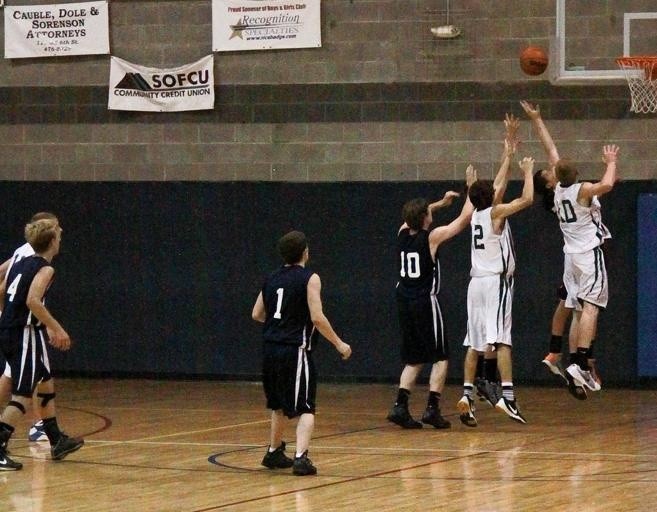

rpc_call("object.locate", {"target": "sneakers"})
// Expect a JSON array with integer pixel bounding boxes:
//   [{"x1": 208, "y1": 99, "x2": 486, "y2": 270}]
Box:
[
  {"x1": 29, "y1": 425, "x2": 49, "y2": 441},
  {"x1": 261, "y1": 441, "x2": 293, "y2": 468},
  {"x1": 291, "y1": 450, "x2": 316, "y2": 476},
  {"x1": 421, "y1": 406, "x2": 451, "y2": 429},
  {"x1": 495, "y1": 396, "x2": 527, "y2": 424},
  {"x1": 51, "y1": 435, "x2": 84, "y2": 461},
  {"x1": 477, "y1": 377, "x2": 500, "y2": 408},
  {"x1": 457, "y1": 394, "x2": 478, "y2": 427},
  {"x1": 387, "y1": 403, "x2": 422, "y2": 429},
  {"x1": 0, "y1": 452, "x2": 22, "y2": 471},
  {"x1": 541, "y1": 352, "x2": 602, "y2": 401}
]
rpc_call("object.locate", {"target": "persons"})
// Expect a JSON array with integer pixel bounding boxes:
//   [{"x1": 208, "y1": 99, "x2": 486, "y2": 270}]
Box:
[
  {"x1": 456, "y1": 133, "x2": 535, "y2": 427},
  {"x1": 387, "y1": 163, "x2": 478, "y2": 429},
  {"x1": 520, "y1": 100, "x2": 603, "y2": 400},
  {"x1": 0, "y1": 218, "x2": 84, "y2": 471},
  {"x1": 0, "y1": 212, "x2": 63, "y2": 442},
  {"x1": 474, "y1": 111, "x2": 522, "y2": 409},
  {"x1": 540, "y1": 144, "x2": 619, "y2": 392},
  {"x1": 252, "y1": 230, "x2": 352, "y2": 476}
]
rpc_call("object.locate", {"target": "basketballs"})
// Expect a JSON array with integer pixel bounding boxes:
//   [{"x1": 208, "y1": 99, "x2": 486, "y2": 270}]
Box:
[{"x1": 521, "y1": 47, "x2": 548, "y2": 75}]
